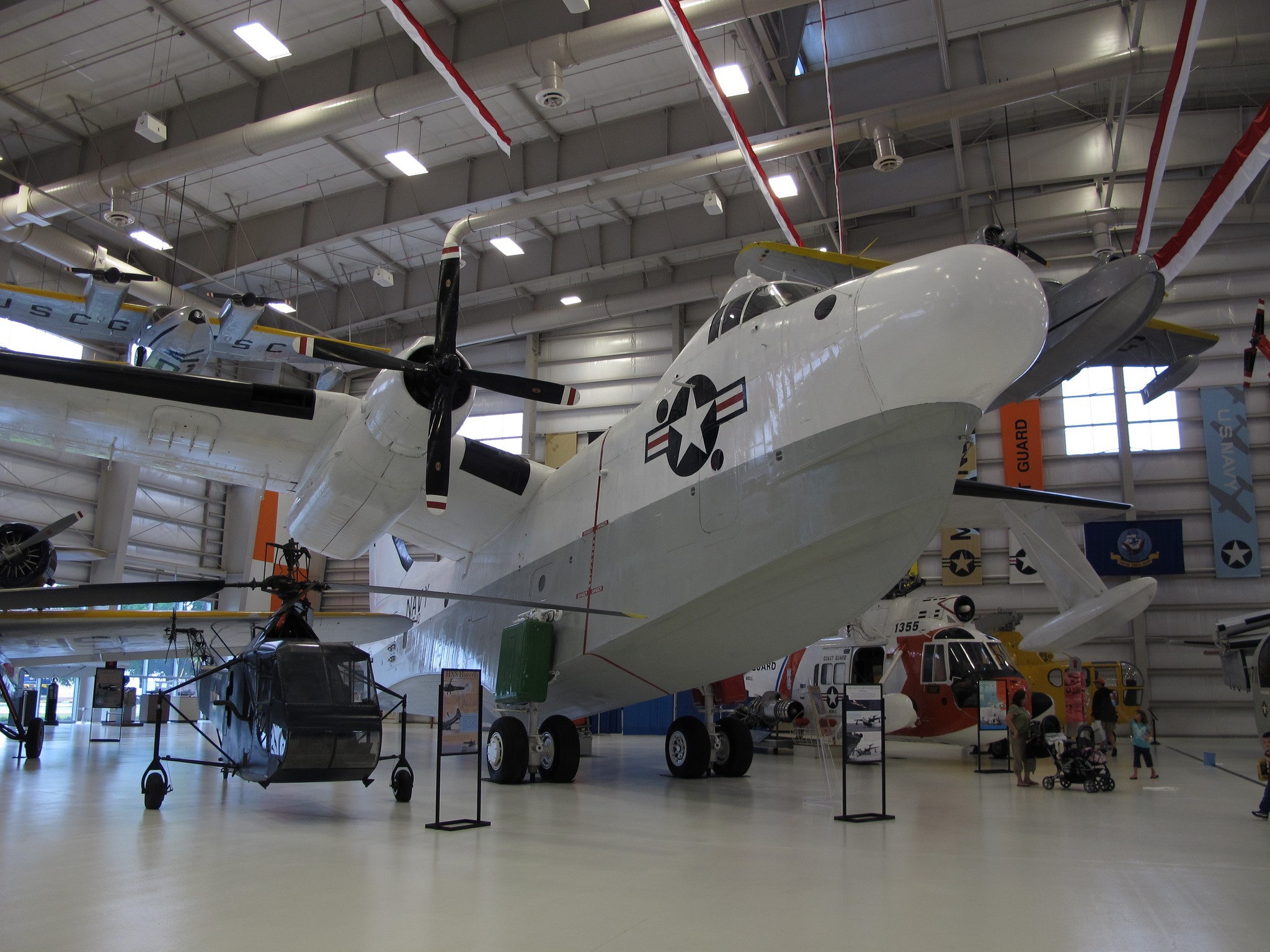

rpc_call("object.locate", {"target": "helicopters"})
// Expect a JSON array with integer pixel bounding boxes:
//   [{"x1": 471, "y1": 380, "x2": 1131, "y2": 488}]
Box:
[{"x1": 0, "y1": 530, "x2": 648, "y2": 812}]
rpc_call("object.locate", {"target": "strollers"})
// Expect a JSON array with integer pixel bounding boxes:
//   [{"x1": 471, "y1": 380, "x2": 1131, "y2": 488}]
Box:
[{"x1": 1036, "y1": 715, "x2": 1116, "y2": 793}]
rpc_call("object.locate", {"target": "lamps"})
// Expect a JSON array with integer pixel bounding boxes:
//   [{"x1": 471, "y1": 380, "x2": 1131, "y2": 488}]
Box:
[
  {"x1": 561, "y1": 275, "x2": 582, "y2": 305},
  {"x1": 490, "y1": 202, "x2": 525, "y2": 256},
  {"x1": 384, "y1": 111, "x2": 430, "y2": 176},
  {"x1": 713, "y1": 24, "x2": 749, "y2": 97},
  {"x1": 234, "y1": 0, "x2": 292, "y2": 61},
  {"x1": 267, "y1": 263, "x2": 296, "y2": 314},
  {"x1": 131, "y1": 187, "x2": 175, "y2": 251},
  {"x1": 768, "y1": 154, "x2": 798, "y2": 198}
]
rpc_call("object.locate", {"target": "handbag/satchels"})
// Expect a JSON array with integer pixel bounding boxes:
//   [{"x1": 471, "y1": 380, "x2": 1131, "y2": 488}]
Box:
[
  {"x1": 1082, "y1": 747, "x2": 1108, "y2": 765},
  {"x1": 1087, "y1": 720, "x2": 1106, "y2": 744},
  {"x1": 1045, "y1": 732, "x2": 1067, "y2": 760}
]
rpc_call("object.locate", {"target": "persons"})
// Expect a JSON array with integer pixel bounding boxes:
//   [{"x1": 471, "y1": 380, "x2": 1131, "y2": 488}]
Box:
[
  {"x1": 1005, "y1": 689, "x2": 1039, "y2": 786},
  {"x1": 1251, "y1": 732, "x2": 1270, "y2": 818},
  {"x1": 1129, "y1": 709, "x2": 1159, "y2": 779},
  {"x1": 1090, "y1": 678, "x2": 1118, "y2": 757}
]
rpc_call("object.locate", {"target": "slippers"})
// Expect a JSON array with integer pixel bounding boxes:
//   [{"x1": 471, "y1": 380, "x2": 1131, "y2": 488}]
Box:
[
  {"x1": 1028, "y1": 780, "x2": 1039, "y2": 785},
  {"x1": 1017, "y1": 783, "x2": 1030, "y2": 787},
  {"x1": 1130, "y1": 776, "x2": 1137, "y2": 779},
  {"x1": 1150, "y1": 774, "x2": 1159, "y2": 779}
]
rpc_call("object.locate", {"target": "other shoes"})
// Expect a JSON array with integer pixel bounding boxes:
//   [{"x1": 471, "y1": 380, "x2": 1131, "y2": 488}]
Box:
[
  {"x1": 1099, "y1": 748, "x2": 1107, "y2": 752},
  {"x1": 1251, "y1": 811, "x2": 1269, "y2": 820},
  {"x1": 1112, "y1": 746, "x2": 1117, "y2": 757}
]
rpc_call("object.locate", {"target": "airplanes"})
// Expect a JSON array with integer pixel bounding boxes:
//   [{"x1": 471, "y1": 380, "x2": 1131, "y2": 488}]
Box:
[
  {"x1": 685, "y1": 566, "x2": 1032, "y2": 766},
  {"x1": 0, "y1": 181, "x2": 1140, "y2": 786},
  {"x1": 0, "y1": 263, "x2": 392, "y2": 366},
  {"x1": 731, "y1": 189, "x2": 1223, "y2": 420}
]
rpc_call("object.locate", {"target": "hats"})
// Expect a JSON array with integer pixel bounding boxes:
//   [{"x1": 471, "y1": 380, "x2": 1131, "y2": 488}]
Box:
[{"x1": 1093, "y1": 678, "x2": 1105, "y2": 684}]
